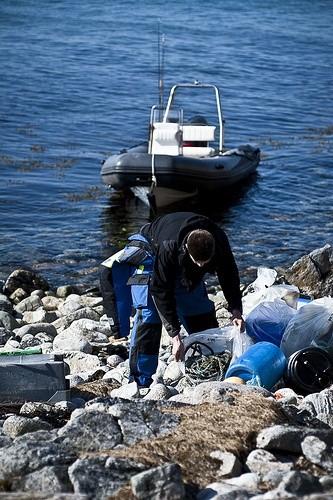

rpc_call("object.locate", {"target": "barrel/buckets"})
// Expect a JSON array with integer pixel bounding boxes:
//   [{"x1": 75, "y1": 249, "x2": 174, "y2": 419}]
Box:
[
  {"x1": 283, "y1": 346, "x2": 333, "y2": 397},
  {"x1": 226, "y1": 341, "x2": 287, "y2": 390}
]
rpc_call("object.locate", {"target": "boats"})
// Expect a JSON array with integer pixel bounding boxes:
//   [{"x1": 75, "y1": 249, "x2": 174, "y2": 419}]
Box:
[{"x1": 100, "y1": 16, "x2": 261, "y2": 210}]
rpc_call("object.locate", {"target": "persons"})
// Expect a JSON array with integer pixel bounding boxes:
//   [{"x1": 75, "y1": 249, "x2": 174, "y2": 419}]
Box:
[{"x1": 129, "y1": 212, "x2": 245, "y2": 398}]
